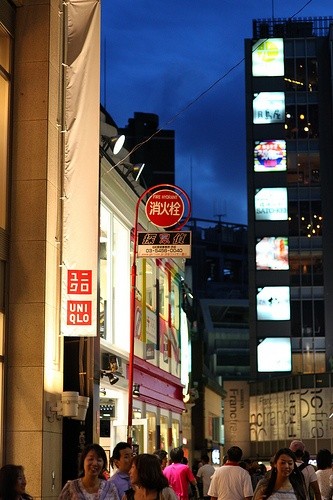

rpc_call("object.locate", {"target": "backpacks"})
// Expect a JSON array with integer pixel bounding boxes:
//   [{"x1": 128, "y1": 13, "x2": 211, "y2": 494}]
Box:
[{"x1": 289, "y1": 462, "x2": 311, "y2": 500}]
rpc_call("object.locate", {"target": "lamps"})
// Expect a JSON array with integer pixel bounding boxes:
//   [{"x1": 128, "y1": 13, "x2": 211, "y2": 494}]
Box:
[
  {"x1": 47, "y1": 392, "x2": 91, "y2": 422},
  {"x1": 122, "y1": 162, "x2": 146, "y2": 181},
  {"x1": 101, "y1": 135, "x2": 125, "y2": 154},
  {"x1": 133, "y1": 384, "x2": 140, "y2": 398},
  {"x1": 101, "y1": 372, "x2": 119, "y2": 385}
]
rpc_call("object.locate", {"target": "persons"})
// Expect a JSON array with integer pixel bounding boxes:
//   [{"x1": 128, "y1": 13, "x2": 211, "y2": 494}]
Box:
[
  {"x1": 59, "y1": 444, "x2": 119, "y2": 500},
  {"x1": 0, "y1": 464, "x2": 33, "y2": 500},
  {"x1": 109, "y1": 440, "x2": 333, "y2": 500}
]
